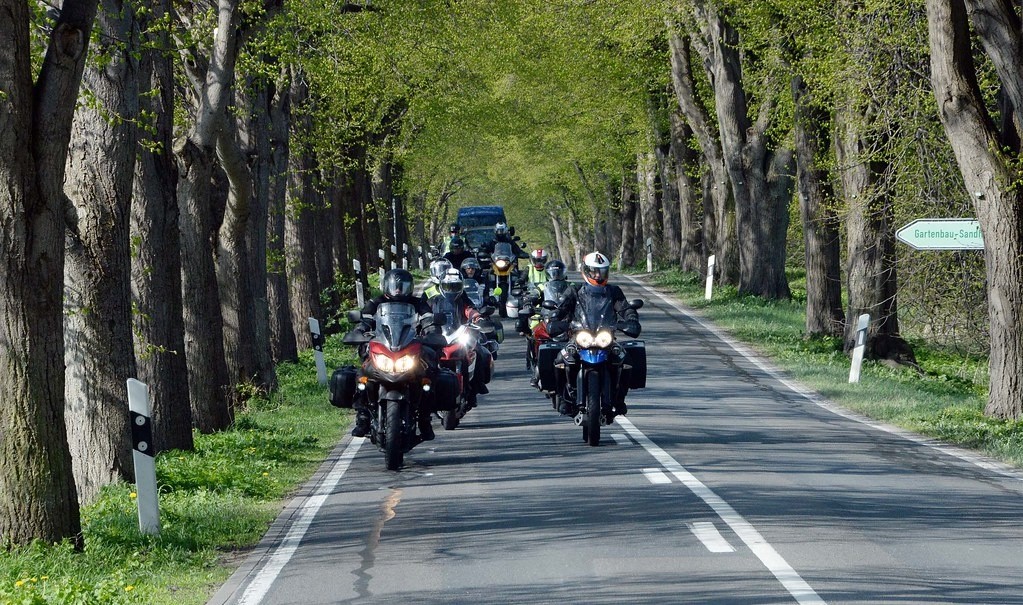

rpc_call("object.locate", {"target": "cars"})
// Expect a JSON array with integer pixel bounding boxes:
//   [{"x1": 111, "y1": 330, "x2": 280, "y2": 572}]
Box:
[{"x1": 465, "y1": 226, "x2": 520, "y2": 264}]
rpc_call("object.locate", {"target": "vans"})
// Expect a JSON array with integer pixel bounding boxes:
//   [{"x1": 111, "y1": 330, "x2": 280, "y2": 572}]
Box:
[{"x1": 450, "y1": 205, "x2": 515, "y2": 236}]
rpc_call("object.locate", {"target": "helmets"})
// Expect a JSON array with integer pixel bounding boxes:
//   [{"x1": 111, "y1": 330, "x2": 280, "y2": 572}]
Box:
[
  {"x1": 382, "y1": 268, "x2": 413, "y2": 302},
  {"x1": 545, "y1": 260, "x2": 567, "y2": 282},
  {"x1": 494, "y1": 222, "x2": 509, "y2": 236},
  {"x1": 430, "y1": 258, "x2": 453, "y2": 284},
  {"x1": 449, "y1": 238, "x2": 463, "y2": 253},
  {"x1": 529, "y1": 249, "x2": 549, "y2": 271},
  {"x1": 439, "y1": 267, "x2": 465, "y2": 303},
  {"x1": 449, "y1": 222, "x2": 460, "y2": 233},
  {"x1": 461, "y1": 257, "x2": 482, "y2": 278},
  {"x1": 581, "y1": 252, "x2": 610, "y2": 287}
]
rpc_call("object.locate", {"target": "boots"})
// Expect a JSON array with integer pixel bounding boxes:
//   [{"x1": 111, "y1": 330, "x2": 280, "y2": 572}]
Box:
[
  {"x1": 419, "y1": 409, "x2": 436, "y2": 441},
  {"x1": 352, "y1": 411, "x2": 372, "y2": 437}
]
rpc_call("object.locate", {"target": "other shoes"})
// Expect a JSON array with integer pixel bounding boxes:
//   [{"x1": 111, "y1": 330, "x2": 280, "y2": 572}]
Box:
[
  {"x1": 469, "y1": 387, "x2": 478, "y2": 407},
  {"x1": 615, "y1": 400, "x2": 627, "y2": 415},
  {"x1": 529, "y1": 376, "x2": 538, "y2": 386},
  {"x1": 478, "y1": 384, "x2": 489, "y2": 394}
]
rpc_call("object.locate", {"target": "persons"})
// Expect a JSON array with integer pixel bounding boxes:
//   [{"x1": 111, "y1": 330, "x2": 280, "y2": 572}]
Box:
[
  {"x1": 352, "y1": 223, "x2": 497, "y2": 441},
  {"x1": 473, "y1": 222, "x2": 641, "y2": 415}
]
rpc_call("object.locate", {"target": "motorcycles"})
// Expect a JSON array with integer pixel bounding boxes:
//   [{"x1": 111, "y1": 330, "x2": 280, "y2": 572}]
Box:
[
  {"x1": 533, "y1": 298, "x2": 646, "y2": 445},
  {"x1": 412, "y1": 241, "x2": 577, "y2": 430},
  {"x1": 328, "y1": 305, "x2": 458, "y2": 470}
]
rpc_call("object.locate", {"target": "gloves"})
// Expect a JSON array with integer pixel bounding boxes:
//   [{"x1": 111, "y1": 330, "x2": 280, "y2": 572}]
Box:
[
  {"x1": 488, "y1": 295, "x2": 497, "y2": 305},
  {"x1": 623, "y1": 318, "x2": 641, "y2": 338}
]
